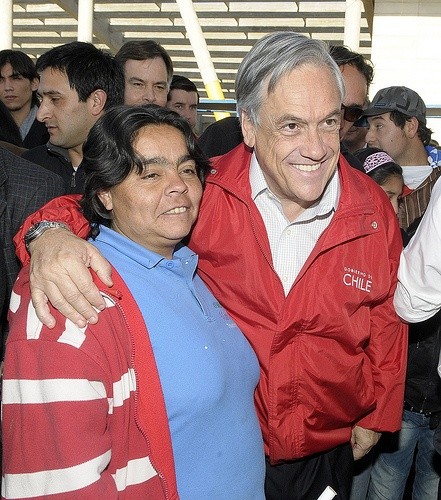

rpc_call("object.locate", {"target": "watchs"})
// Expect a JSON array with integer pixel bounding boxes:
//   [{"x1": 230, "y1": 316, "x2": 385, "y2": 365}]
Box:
[{"x1": 24, "y1": 221, "x2": 71, "y2": 255}]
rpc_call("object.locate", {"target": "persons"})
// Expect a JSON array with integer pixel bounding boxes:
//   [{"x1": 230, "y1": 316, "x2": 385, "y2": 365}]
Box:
[
  {"x1": 1, "y1": 104, "x2": 266, "y2": 500},
  {"x1": 197, "y1": 45, "x2": 441, "y2": 500},
  {"x1": 393, "y1": 175, "x2": 441, "y2": 378},
  {"x1": 13, "y1": 31, "x2": 408, "y2": 500},
  {"x1": 0, "y1": 40, "x2": 200, "y2": 379}
]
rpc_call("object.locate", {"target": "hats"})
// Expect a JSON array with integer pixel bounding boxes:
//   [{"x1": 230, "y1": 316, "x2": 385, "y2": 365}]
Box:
[{"x1": 365, "y1": 85, "x2": 427, "y2": 126}]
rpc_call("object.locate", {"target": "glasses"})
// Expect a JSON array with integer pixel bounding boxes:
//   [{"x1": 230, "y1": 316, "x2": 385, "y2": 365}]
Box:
[{"x1": 341, "y1": 101, "x2": 364, "y2": 123}]
